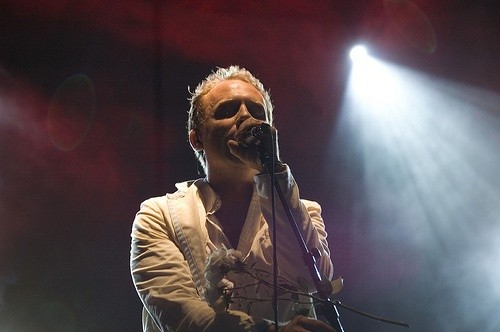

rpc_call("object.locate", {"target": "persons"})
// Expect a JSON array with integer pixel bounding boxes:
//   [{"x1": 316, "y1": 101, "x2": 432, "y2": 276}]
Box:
[{"x1": 130, "y1": 66, "x2": 338, "y2": 332}]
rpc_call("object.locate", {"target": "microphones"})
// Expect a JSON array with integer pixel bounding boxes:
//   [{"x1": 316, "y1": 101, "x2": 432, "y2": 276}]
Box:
[{"x1": 236, "y1": 122, "x2": 270, "y2": 146}]
